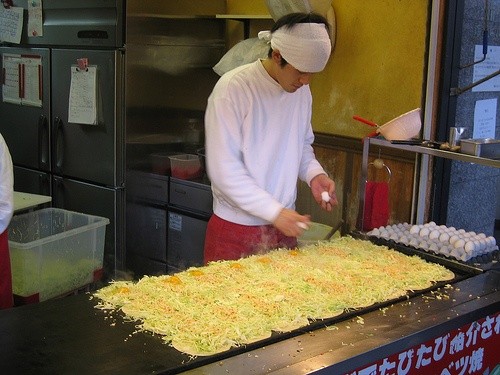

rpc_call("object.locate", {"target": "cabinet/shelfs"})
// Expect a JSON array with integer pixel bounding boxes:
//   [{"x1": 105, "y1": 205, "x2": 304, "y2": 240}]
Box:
[
  {"x1": 358, "y1": 137, "x2": 500, "y2": 265},
  {"x1": 0, "y1": 44, "x2": 125, "y2": 281},
  {"x1": 167, "y1": 178, "x2": 212, "y2": 267}
]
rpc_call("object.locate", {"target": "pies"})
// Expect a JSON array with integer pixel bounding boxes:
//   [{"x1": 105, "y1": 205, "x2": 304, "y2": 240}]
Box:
[{"x1": 122, "y1": 271, "x2": 455, "y2": 356}]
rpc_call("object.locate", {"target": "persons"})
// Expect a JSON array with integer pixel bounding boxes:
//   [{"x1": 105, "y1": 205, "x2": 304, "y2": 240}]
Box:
[
  {"x1": 0, "y1": 133, "x2": 14, "y2": 310},
  {"x1": 204, "y1": 12, "x2": 338, "y2": 267}
]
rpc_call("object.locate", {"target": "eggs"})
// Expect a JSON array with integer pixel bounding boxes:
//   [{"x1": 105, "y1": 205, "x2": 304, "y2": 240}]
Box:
[{"x1": 410, "y1": 221, "x2": 496, "y2": 253}]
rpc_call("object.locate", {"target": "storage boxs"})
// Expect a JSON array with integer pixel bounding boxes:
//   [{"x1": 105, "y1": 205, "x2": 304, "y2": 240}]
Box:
[{"x1": 7, "y1": 208, "x2": 110, "y2": 305}]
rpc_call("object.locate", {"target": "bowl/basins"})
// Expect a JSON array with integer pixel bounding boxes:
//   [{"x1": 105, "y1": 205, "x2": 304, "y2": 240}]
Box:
[
  {"x1": 375, "y1": 107, "x2": 422, "y2": 141},
  {"x1": 168, "y1": 154, "x2": 204, "y2": 180}
]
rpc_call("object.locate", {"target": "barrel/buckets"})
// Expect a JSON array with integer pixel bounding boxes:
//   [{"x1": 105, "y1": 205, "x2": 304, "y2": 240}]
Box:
[{"x1": 294, "y1": 221, "x2": 341, "y2": 245}]
